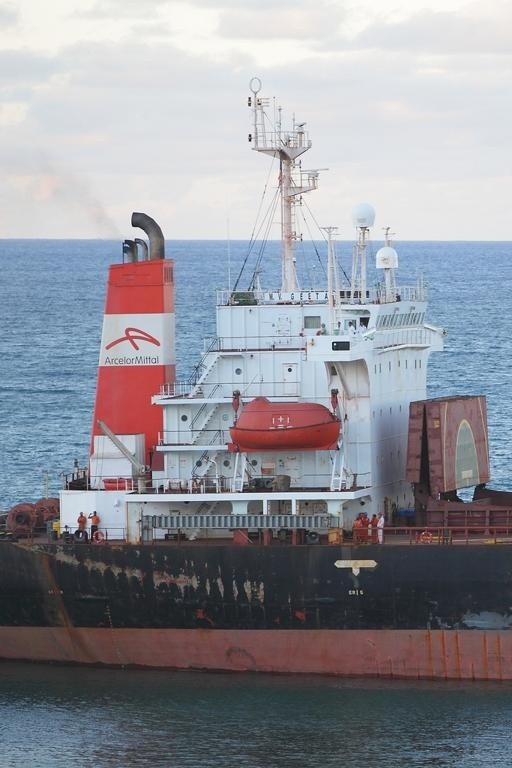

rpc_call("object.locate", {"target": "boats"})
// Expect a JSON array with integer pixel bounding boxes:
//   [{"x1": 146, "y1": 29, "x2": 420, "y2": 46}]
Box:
[{"x1": 0, "y1": 76, "x2": 512, "y2": 679}]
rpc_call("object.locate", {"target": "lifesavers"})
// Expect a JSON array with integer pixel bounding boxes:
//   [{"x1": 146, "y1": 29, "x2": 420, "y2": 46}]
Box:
[
  {"x1": 74, "y1": 530, "x2": 88, "y2": 543},
  {"x1": 306, "y1": 532, "x2": 320, "y2": 545},
  {"x1": 420, "y1": 532, "x2": 432, "y2": 543},
  {"x1": 93, "y1": 532, "x2": 104, "y2": 543},
  {"x1": 192, "y1": 480, "x2": 198, "y2": 491}
]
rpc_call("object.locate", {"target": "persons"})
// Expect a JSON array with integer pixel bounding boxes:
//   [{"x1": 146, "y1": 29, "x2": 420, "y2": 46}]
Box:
[
  {"x1": 353, "y1": 511, "x2": 386, "y2": 543},
  {"x1": 78, "y1": 512, "x2": 86, "y2": 533},
  {"x1": 88, "y1": 510, "x2": 101, "y2": 544}
]
